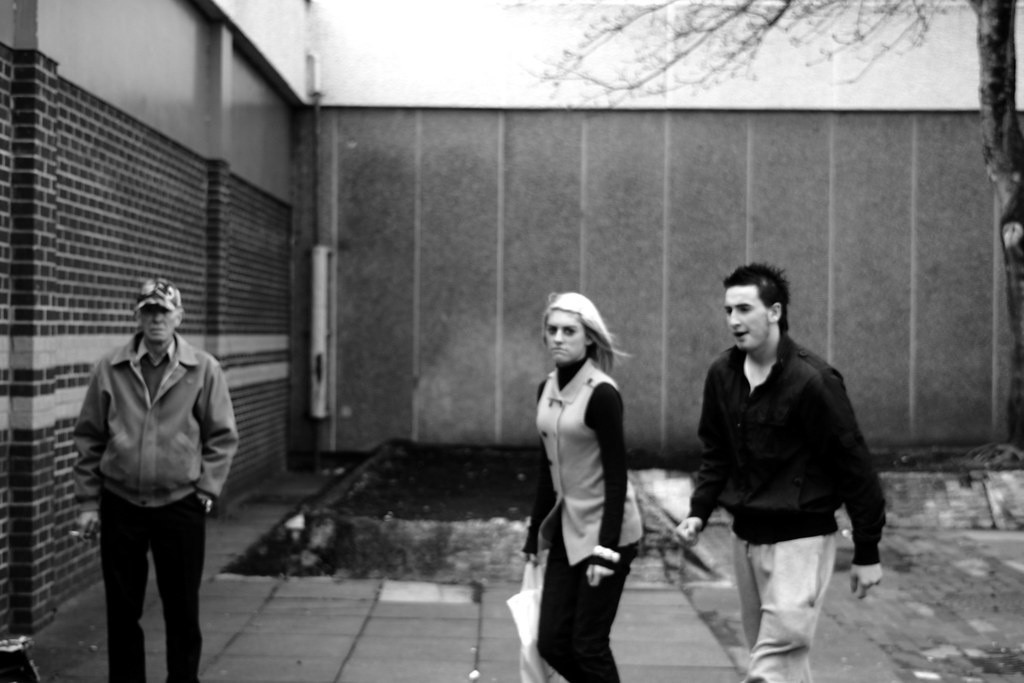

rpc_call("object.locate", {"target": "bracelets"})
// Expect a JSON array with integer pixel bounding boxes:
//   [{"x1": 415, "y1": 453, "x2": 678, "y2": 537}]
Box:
[{"x1": 592, "y1": 545, "x2": 620, "y2": 563}]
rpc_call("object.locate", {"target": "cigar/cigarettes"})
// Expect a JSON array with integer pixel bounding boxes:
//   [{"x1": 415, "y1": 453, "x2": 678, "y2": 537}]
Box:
[{"x1": 70, "y1": 531, "x2": 79, "y2": 537}]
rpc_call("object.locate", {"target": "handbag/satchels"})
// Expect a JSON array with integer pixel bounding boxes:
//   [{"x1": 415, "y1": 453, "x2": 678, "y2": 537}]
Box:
[{"x1": 506, "y1": 562, "x2": 569, "y2": 683}]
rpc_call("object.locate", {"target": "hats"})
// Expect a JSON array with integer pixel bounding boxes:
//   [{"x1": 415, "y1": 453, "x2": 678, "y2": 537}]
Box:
[{"x1": 134, "y1": 278, "x2": 181, "y2": 311}]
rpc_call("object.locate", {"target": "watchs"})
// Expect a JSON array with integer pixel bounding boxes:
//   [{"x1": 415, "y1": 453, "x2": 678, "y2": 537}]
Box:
[{"x1": 199, "y1": 496, "x2": 212, "y2": 513}]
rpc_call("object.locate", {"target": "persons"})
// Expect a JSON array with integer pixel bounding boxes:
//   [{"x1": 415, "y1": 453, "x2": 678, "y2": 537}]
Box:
[
  {"x1": 522, "y1": 288, "x2": 642, "y2": 683},
  {"x1": 72, "y1": 278, "x2": 239, "y2": 683},
  {"x1": 674, "y1": 263, "x2": 886, "y2": 683}
]
